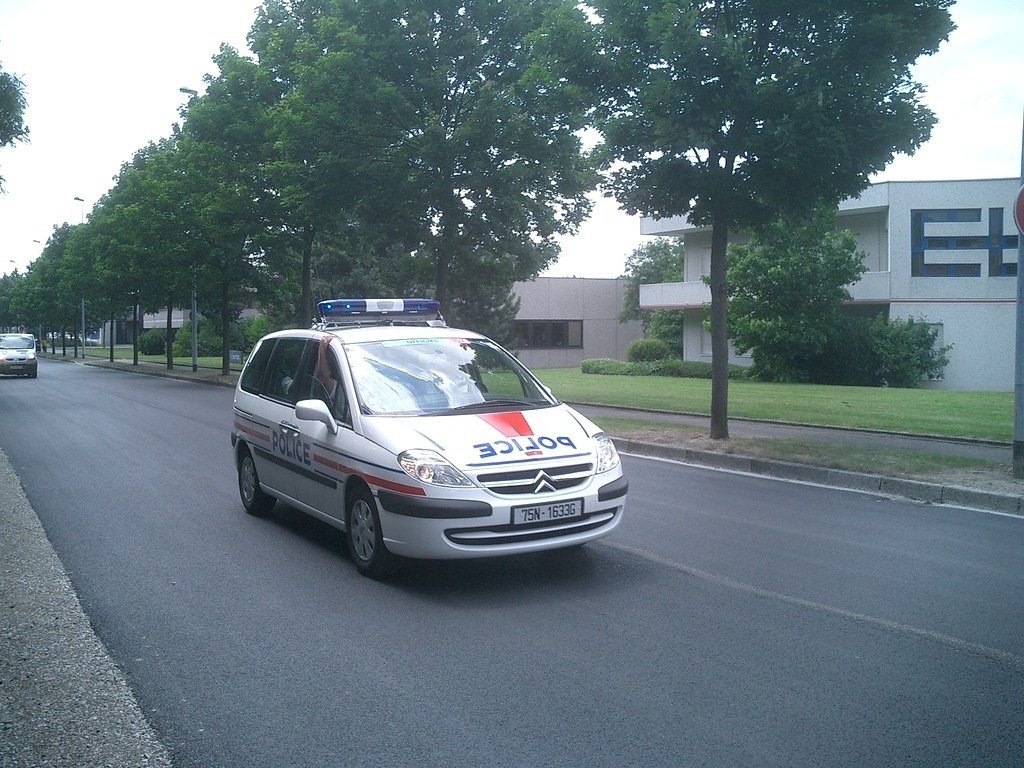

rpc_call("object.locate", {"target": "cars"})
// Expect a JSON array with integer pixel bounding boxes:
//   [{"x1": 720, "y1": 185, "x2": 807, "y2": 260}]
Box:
[{"x1": 230, "y1": 298, "x2": 629, "y2": 578}]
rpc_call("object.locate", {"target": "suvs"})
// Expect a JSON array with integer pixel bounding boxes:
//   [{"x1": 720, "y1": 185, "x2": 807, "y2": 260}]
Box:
[{"x1": 0, "y1": 333, "x2": 39, "y2": 379}]
